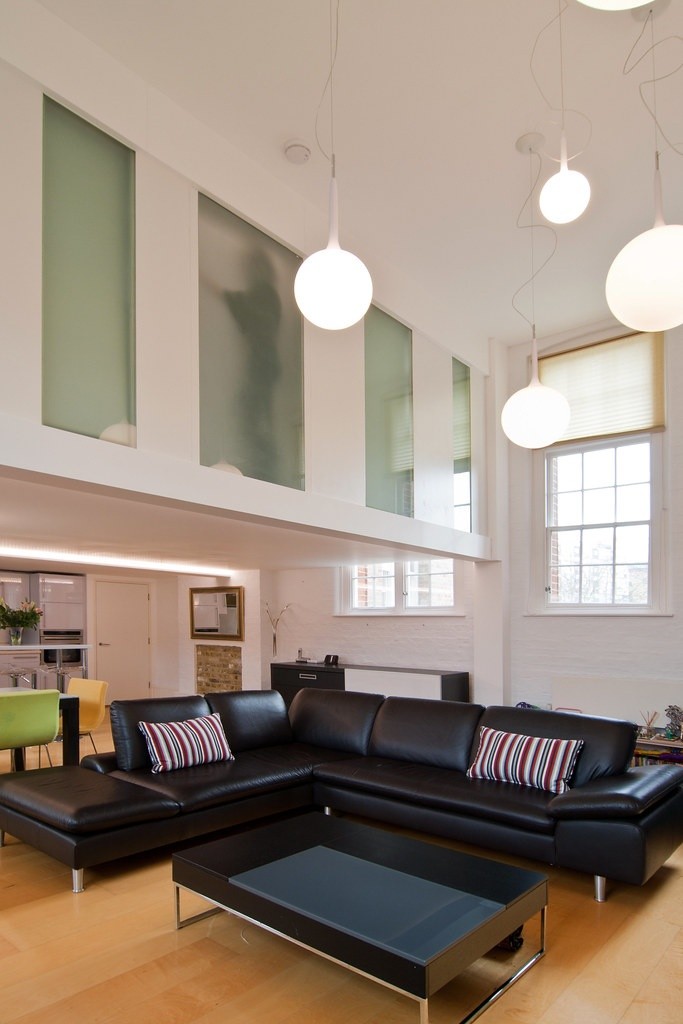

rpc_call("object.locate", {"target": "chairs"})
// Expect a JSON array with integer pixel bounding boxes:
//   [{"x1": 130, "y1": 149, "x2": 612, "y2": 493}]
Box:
[
  {"x1": 0, "y1": 689, "x2": 60, "y2": 774},
  {"x1": 39, "y1": 677, "x2": 108, "y2": 768}
]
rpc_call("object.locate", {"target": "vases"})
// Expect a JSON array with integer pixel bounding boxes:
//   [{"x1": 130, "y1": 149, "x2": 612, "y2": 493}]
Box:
[
  {"x1": 271, "y1": 626, "x2": 279, "y2": 662},
  {"x1": 8, "y1": 627, "x2": 23, "y2": 646}
]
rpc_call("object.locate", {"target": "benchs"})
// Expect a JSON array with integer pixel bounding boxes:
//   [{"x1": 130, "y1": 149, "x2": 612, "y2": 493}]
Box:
[
  {"x1": 311, "y1": 696, "x2": 683, "y2": 903},
  {"x1": 0, "y1": 689, "x2": 385, "y2": 894}
]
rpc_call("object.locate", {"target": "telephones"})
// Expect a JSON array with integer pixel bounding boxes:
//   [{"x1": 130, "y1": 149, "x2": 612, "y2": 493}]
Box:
[{"x1": 295, "y1": 648, "x2": 310, "y2": 663}]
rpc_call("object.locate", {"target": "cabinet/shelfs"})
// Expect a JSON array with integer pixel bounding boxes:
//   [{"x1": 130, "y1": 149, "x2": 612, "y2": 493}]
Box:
[{"x1": 269, "y1": 661, "x2": 468, "y2": 708}]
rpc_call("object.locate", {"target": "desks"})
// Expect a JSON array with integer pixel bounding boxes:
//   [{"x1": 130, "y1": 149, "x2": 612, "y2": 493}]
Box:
[
  {"x1": 0, "y1": 687, "x2": 80, "y2": 771},
  {"x1": 0, "y1": 644, "x2": 92, "y2": 744},
  {"x1": 635, "y1": 737, "x2": 683, "y2": 749}
]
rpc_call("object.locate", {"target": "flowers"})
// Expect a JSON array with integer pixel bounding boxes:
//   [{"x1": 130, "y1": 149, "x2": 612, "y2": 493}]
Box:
[{"x1": 0, "y1": 597, "x2": 44, "y2": 644}]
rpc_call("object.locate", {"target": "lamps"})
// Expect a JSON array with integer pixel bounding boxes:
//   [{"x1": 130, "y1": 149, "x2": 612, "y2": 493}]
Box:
[
  {"x1": 284, "y1": 136, "x2": 313, "y2": 164},
  {"x1": 604, "y1": 0, "x2": 683, "y2": 334},
  {"x1": 210, "y1": 458, "x2": 243, "y2": 478},
  {"x1": 499, "y1": 132, "x2": 564, "y2": 448},
  {"x1": 529, "y1": 0, "x2": 592, "y2": 226},
  {"x1": 100, "y1": 417, "x2": 138, "y2": 449},
  {"x1": 293, "y1": 0, "x2": 374, "y2": 330}
]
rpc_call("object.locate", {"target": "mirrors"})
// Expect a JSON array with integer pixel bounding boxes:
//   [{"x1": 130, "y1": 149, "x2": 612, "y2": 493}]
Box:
[{"x1": 189, "y1": 586, "x2": 246, "y2": 644}]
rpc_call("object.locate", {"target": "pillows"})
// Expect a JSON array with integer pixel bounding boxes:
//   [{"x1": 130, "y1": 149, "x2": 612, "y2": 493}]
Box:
[
  {"x1": 465, "y1": 725, "x2": 584, "y2": 795},
  {"x1": 136, "y1": 712, "x2": 236, "y2": 774}
]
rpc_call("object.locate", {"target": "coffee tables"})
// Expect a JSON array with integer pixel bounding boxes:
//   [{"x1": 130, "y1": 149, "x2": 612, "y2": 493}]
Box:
[{"x1": 171, "y1": 811, "x2": 551, "y2": 1024}]
